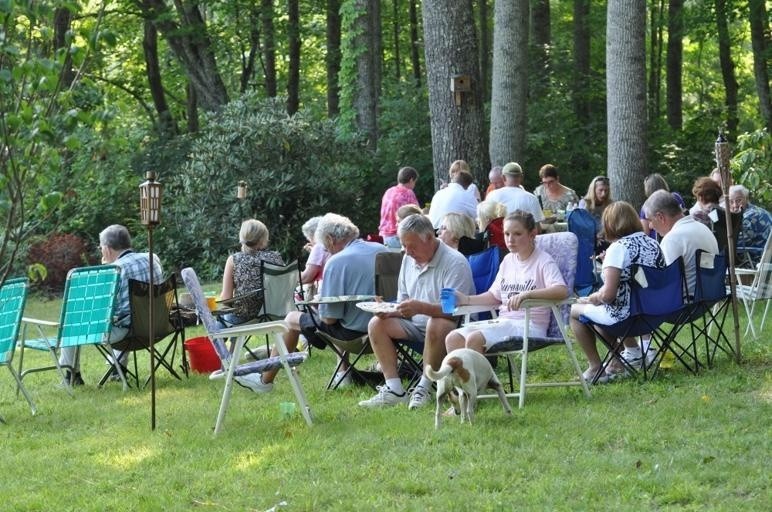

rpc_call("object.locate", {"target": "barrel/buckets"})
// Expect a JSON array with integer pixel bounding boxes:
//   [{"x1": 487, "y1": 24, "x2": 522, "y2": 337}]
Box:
[{"x1": 183, "y1": 336, "x2": 221, "y2": 372}]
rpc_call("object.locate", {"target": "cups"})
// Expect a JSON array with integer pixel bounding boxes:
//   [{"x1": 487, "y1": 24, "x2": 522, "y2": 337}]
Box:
[
  {"x1": 299, "y1": 284, "x2": 313, "y2": 302},
  {"x1": 203, "y1": 291, "x2": 216, "y2": 311},
  {"x1": 707, "y1": 209, "x2": 719, "y2": 223},
  {"x1": 180, "y1": 293, "x2": 192, "y2": 305},
  {"x1": 558, "y1": 210, "x2": 565, "y2": 222},
  {"x1": 441, "y1": 288, "x2": 460, "y2": 313}
]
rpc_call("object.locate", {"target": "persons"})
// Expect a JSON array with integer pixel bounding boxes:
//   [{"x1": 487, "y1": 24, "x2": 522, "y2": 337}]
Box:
[
  {"x1": 59, "y1": 223, "x2": 163, "y2": 387},
  {"x1": 357, "y1": 214, "x2": 477, "y2": 410},
  {"x1": 445, "y1": 210, "x2": 568, "y2": 417},
  {"x1": 294, "y1": 215, "x2": 332, "y2": 311},
  {"x1": 234, "y1": 212, "x2": 391, "y2": 396},
  {"x1": 379, "y1": 161, "x2": 730, "y2": 250},
  {"x1": 729, "y1": 183, "x2": 772, "y2": 267},
  {"x1": 570, "y1": 200, "x2": 666, "y2": 385},
  {"x1": 604, "y1": 189, "x2": 719, "y2": 372},
  {"x1": 212, "y1": 219, "x2": 284, "y2": 340}
]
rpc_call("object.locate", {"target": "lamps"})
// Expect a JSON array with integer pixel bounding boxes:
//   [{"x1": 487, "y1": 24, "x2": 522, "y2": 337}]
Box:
[
  {"x1": 139, "y1": 170, "x2": 163, "y2": 430},
  {"x1": 715, "y1": 130, "x2": 741, "y2": 364},
  {"x1": 237, "y1": 180, "x2": 247, "y2": 222}
]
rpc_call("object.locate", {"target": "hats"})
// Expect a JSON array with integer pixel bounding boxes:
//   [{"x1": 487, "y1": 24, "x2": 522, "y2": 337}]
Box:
[{"x1": 502, "y1": 162, "x2": 522, "y2": 175}]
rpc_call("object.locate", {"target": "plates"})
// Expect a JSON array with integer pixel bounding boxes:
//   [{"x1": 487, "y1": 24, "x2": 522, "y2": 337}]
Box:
[{"x1": 355, "y1": 301, "x2": 398, "y2": 314}]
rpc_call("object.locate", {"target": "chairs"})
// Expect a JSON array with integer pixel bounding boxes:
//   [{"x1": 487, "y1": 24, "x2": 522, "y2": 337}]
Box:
[
  {"x1": 215, "y1": 259, "x2": 306, "y2": 361},
  {"x1": 182, "y1": 267, "x2": 313, "y2": 434},
  {"x1": 98, "y1": 272, "x2": 189, "y2": 390},
  {"x1": 315, "y1": 251, "x2": 404, "y2": 391},
  {"x1": 579, "y1": 205, "x2": 772, "y2": 384},
  {"x1": 452, "y1": 232, "x2": 593, "y2": 408},
  {"x1": 566, "y1": 209, "x2": 598, "y2": 297},
  {"x1": 0, "y1": 276, "x2": 37, "y2": 424},
  {"x1": 395, "y1": 243, "x2": 515, "y2": 394},
  {"x1": 16, "y1": 264, "x2": 127, "y2": 398}
]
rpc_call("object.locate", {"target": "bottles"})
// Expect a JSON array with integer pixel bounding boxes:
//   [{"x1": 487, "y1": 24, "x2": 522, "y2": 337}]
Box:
[
  {"x1": 566, "y1": 202, "x2": 573, "y2": 220},
  {"x1": 659, "y1": 332, "x2": 676, "y2": 368},
  {"x1": 573, "y1": 203, "x2": 577, "y2": 209}
]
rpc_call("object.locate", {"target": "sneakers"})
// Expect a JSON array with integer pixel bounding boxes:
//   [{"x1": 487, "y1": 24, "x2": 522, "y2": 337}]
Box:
[
  {"x1": 334, "y1": 371, "x2": 351, "y2": 386},
  {"x1": 358, "y1": 384, "x2": 408, "y2": 406},
  {"x1": 408, "y1": 385, "x2": 432, "y2": 411},
  {"x1": 234, "y1": 373, "x2": 273, "y2": 393},
  {"x1": 579, "y1": 347, "x2": 658, "y2": 383}
]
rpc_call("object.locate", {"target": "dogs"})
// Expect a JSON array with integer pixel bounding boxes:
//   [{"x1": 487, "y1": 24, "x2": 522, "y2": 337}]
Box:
[{"x1": 424, "y1": 347, "x2": 513, "y2": 429}]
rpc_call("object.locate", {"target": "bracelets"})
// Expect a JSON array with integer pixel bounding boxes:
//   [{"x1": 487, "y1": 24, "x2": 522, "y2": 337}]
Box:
[{"x1": 468, "y1": 295, "x2": 471, "y2": 305}]
